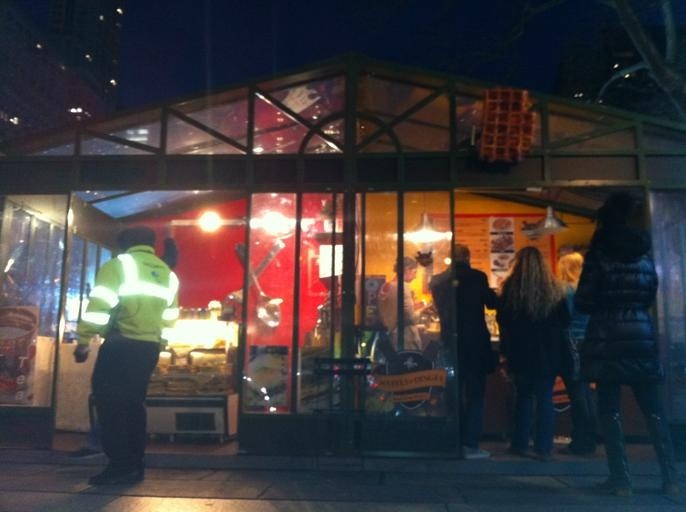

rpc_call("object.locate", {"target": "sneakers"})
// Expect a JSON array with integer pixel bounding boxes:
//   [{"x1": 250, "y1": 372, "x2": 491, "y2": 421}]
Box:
[
  {"x1": 87, "y1": 461, "x2": 145, "y2": 485},
  {"x1": 558, "y1": 438, "x2": 597, "y2": 456},
  {"x1": 464, "y1": 446, "x2": 490, "y2": 460},
  {"x1": 664, "y1": 484, "x2": 681, "y2": 500},
  {"x1": 505, "y1": 444, "x2": 554, "y2": 463},
  {"x1": 602, "y1": 484, "x2": 632, "y2": 497}
]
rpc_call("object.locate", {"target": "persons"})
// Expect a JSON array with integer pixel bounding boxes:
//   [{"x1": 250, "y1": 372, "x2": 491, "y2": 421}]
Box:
[
  {"x1": 376, "y1": 255, "x2": 430, "y2": 354},
  {"x1": 550, "y1": 251, "x2": 601, "y2": 453},
  {"x1": 432, "y1": 243, "x2": 500, "y2": 461},
  {"x1": 155, "y1": 221, "x2": 178, "y2": 271},
  {"x1": 70, "y1": 226, "x2": 182, "y2": 485},
  {"x1": 496, "y1": 243, "x2": 572, "y2": 460},
  {"x1": 572, "y1": 189, "x2": 682, "y2": 498}
]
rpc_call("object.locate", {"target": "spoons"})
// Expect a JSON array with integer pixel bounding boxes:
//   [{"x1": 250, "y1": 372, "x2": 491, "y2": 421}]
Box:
[{"x1": 221, "y1": 239, "x2": 286, "y2": 328}]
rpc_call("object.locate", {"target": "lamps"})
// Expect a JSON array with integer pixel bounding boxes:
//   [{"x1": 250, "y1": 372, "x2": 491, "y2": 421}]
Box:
[
  {"x1": 534, "y1": 206, "x2": 570, "y2": 234},
  {"x1": 394, "y1": 212, "x2": 452, "y2": 244}
]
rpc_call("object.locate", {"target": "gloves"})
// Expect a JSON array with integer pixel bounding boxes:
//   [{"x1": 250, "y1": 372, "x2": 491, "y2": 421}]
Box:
[{"x1": 74, "y1": 343, "x2": 88, "y2": 362}]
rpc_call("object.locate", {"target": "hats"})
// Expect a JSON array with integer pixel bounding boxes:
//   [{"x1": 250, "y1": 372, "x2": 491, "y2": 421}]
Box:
[
  {"x1": 119, "y1": 227, "x2": 155, "y2": 252},
  {"x1": 393, "y1": 257, "x2": 417, "y2": 273}
]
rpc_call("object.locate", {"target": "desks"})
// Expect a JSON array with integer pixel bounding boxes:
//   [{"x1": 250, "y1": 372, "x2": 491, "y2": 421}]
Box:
[{"x1": 89, "y1": 391, "x2": 239, "y2": 444}]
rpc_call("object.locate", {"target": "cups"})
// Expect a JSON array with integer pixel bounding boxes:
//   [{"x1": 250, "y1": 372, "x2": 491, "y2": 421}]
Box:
[{"x1": 1, "y1": 307, "x2": 39, "y2": 406}]
